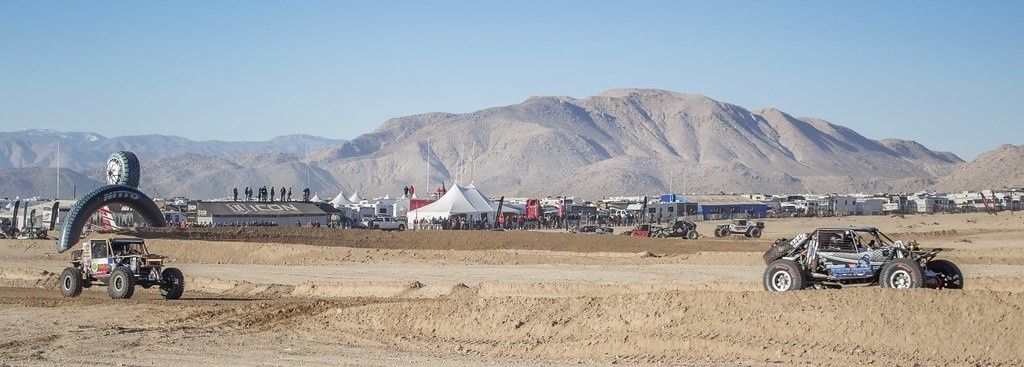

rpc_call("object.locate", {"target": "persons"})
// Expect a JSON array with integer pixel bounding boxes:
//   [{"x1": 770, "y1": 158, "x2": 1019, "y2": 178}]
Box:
[
  {"x1": 128, "y1": 222, "x2": 131, "y2": 226},
  {"x1": 258, "y1": 185, "x2": 267, "y2": 202},
  {"x1": 649, "y1": 213, "x2": 654, "y2": 225},
  {"x1": 287, "y1": 187, "x2": 292, "y2": 201},
  {"x1": 504, "y1": 215, "x2": 581, "y2": 230},
  {"x1": 683, "y1": 211, "x2": 688, "y2": 216},
  {"x1": 828, "y1": 234, "x2": 842, "y2": 249},
  {"x1": 233, "y1": 187, "x2": 238, "y2": 201},
  {"x1": 122, "y1": 223, "x2": 127, "y2": 227},
  {"x1": 586, "y1": 211, "x2": 643, "y2": 226},
  {"x1": 245, "y1": 187, "x2": 253, "y2": 201},
  {"x1": 657, "y1": 211, "x2": 662, "y2": 223},
  {"x1": 302, "y1": 188, "x2": 310, "y2": 202},
  {"x1": 280, "y1": 187, "x2": 286, "y2": 202},
  {"x1": 404, "y1": 185, "x2": 414, "y2": 197},
  {"x1": 413, "y1": 217, "x2": 488, "y2": 230},
  {"x1": 168, "y1": 219, "x2": 279, "y2": 227},
  {"x1": 307, "y1": 220, "x2": 320, "y2": 227},
  {"x1": 271, "y1": 187, "x2": 275, "y2": 201},
  {"x1": 296, "y1": 220, "x2": 301, "y2": 226},
  {"x1": 711, "y1": 207, "x2": 755, "y2": 217},
  {"x1": 690, "y1": 208, "x2": 695, "y2": 214}
]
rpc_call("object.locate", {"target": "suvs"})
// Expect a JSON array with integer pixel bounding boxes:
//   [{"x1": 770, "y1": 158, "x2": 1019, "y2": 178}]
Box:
[
  {"x1": 762, "y1": 224, "x2": 964, "y2": 293},
  {"x1": 59, "y1": 237, "x2": 186, "y2": 300},
  {"x1": 713, "y1": 218, "x2": 765, "y2": 239},
  {"x1": 651, "y1": 218, "x2": 698, "y2": 240},
  {"x1": 0, "y1": 218, "x2": 49, "y2": 240}
]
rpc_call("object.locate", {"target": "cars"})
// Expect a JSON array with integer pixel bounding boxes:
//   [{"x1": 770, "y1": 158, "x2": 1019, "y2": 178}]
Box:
[
  {"x1": 567, "y1": 208, "x2": 633, "y2": 221},
  {"x1": 351, "y1": 212, "x2": 406, "y2": 231},
  {"x1": 573, "y1": 225, "x2": 664, "y2": 237}
]
